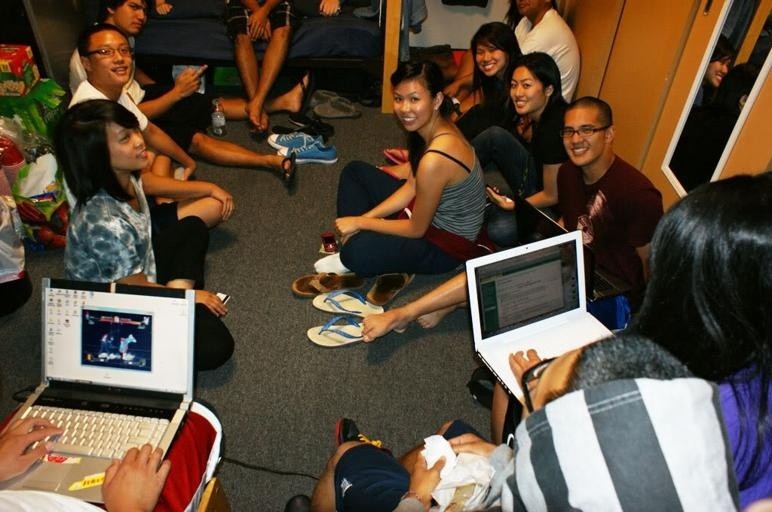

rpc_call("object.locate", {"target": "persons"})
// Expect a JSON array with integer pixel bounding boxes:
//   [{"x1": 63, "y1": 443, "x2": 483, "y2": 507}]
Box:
[
  {"x1": 68, "y1": 2, "x2": 343, "y2": 190},
  {"x1": 1, "y1": 395, "x2": 226, "y2": 512},
  {"x1": 316, "y1": 56, "x2": 485, "y2": 276},
  {"x1": 285, "y1": 338, "x2": 742, "y2": 512},
  {"x1": 669, "y1": 62, "x2": 761, "y2": 196},
  {"x1": 62, "y1": 24, "x2": 236, "y2": 230},
  {"x1": 681, "y1": 32, "x2": 734, "y2": 126},
  {"x1": 375, "y1": 0, "x2": 580, "y2": 251},
  {"x1": 55, "y1": 99, "x2": 235, "y2": 371},
  {"x1": 362, "y1": 96, "x2": 664, "y2": 343},
  {"x1": 436, "y1": 172, "x2": 771, "y2": 511}
]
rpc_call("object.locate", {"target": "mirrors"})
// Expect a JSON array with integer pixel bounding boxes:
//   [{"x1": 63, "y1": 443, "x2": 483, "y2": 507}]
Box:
[{"x1": 661, "y1": 0, "x2": 772, "y2": 199}]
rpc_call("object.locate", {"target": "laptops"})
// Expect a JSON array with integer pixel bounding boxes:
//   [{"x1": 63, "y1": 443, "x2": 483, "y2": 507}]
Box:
[
  {"x1": 2, "y1": 276, "x2": 202, "y2": 506},
  {"x1": 465, "y1": 230, "x2": 611, "y2": 405},
  {"x1": 514, "y1": 191, "x2": 634, "y2": 307}
]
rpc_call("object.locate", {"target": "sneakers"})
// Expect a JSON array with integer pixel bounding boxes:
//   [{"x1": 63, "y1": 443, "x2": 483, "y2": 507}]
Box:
[
  {"x1": 286, "y1": 494, "x2": 312, "y2": 512},
  {"x1": 277, "y1": 142, "x2": 339, "y2": 165},
  {"x1": 335, "y1": 417, "x2": 394, "y2": 457},
  {"x1": 268, "y1": 131, "x2": 326, "y2": 151}
]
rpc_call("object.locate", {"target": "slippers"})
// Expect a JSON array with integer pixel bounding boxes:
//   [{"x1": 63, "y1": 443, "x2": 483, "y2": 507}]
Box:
[
  {"x1": 290, "y1": 270, "x2": 416, "y2": 348},
  {"x1": 372, "y1": 164, "x2": 408, "y2": 184},
  {"x1": 466, "y1": 382, "x2": 494, "y2": 411},
  {"x1": 470, "y1": 366, "x2": 497, "y2": 392},
  {"x1": 383, "y1": 145, "x2": 410, "y2": 165},
  {"x1": 272, "y1": 72, "x2": 362, "y2": 145},
  {"x1": 282, "y1": 152, "x2": 298, "y2": 196}
]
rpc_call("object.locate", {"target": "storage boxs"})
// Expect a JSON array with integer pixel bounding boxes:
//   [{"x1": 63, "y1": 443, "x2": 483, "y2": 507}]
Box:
[
  {"x1": 1, "y1": 77, "x2": 66, "y2": 144},
  {"x1": 1, "y1": 45, "x2": 40, "y2": 96}
]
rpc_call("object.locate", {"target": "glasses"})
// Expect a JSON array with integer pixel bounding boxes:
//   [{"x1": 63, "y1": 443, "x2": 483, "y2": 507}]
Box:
[
  {"x1": 560, "y1": 124, "x2": 611, "y2": 138},
  {"x1": 522, "y1": 357, "x2": 559, "y2": 416},
  {"x1": 85, "y1": 48, "x2": 136, "y2": 60}
]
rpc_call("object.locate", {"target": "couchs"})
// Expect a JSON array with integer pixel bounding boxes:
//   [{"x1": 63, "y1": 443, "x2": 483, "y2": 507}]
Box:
[{"x1": 86, "y1": 0, "x2": 386, "y2": 105}]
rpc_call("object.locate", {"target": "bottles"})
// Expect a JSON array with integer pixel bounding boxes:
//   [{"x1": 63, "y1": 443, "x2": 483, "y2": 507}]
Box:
[{"x1": 211, "y1": 97, "x2": 227, "y2": 136}]
